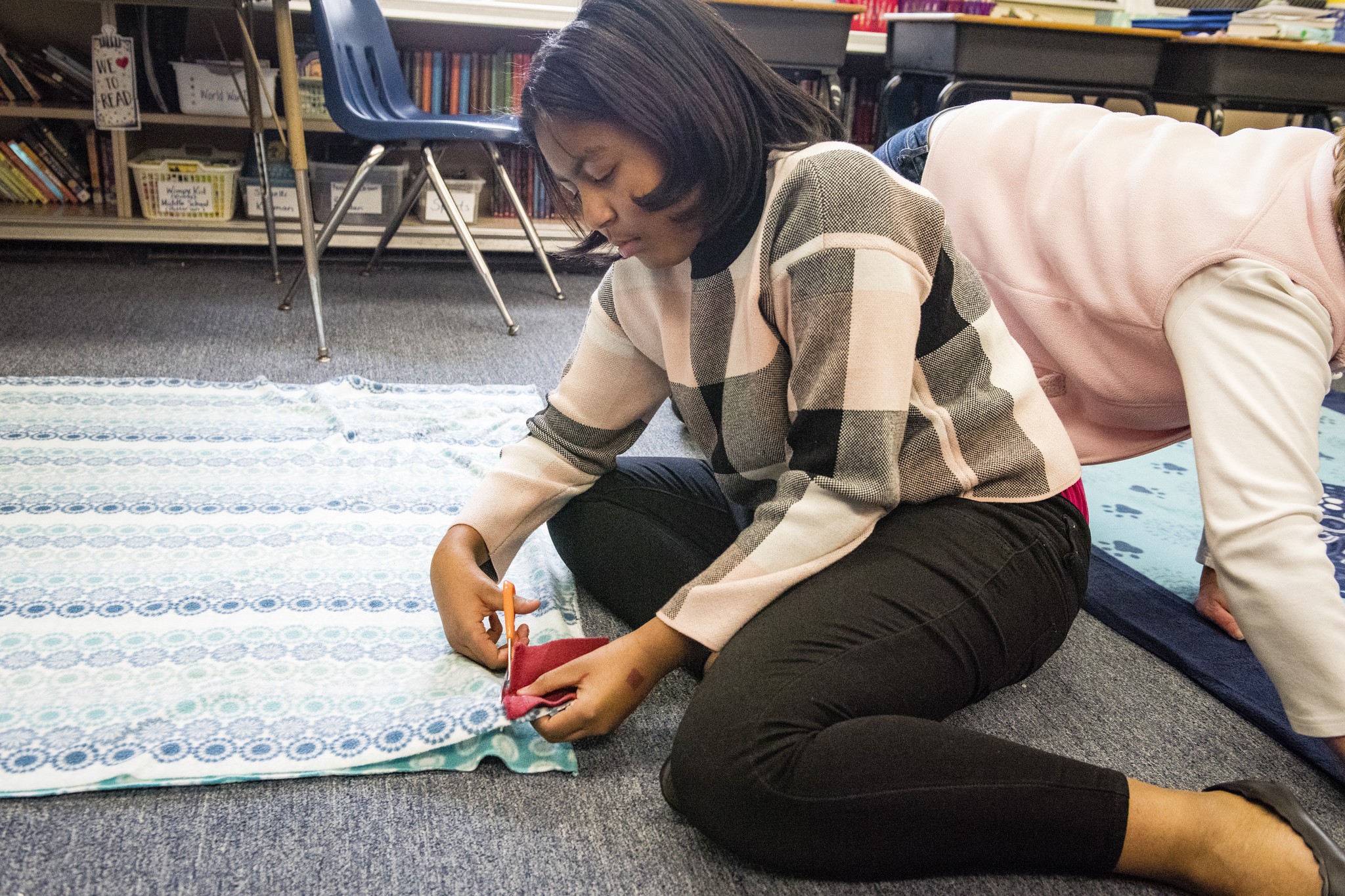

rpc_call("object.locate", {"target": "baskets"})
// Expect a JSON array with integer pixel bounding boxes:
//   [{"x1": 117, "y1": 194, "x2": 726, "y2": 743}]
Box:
[
  {"x1": 127, "y1": 142, "x2": 245, "y2": 222},
  {"x1": 297, "y1": 76, "x2": 336, "y2": 120},
  {"x1": 897, "y1": 0, "x2": 998, "y2": 16},
  {"x1": 837, "y1": 0, "x2": 898, "y2": 33}
]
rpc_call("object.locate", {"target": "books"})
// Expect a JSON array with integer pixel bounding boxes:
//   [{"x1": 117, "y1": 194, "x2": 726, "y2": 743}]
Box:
[
  {"x1": 388, "y1": 39, "x2": 540, "y2": 119},
  {"x1": 0, "y1": 33, "x2": 95, "y2": 109},
  {"x1": 1220, "y1": 0, "x2": 1337, "y2": 46},
  {"x1": 0, "y1": 122, "x2": 121, "y2": 213},
  {"x1": 788, "y1": 67, "x2": 885, "y2": 147},
  {"x1": 484, "y1": 141, "x2": 584, "y2": 222}
]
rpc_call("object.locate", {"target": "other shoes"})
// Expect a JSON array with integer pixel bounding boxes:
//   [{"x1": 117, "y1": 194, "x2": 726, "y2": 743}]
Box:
[{"x1": 1199, "y1": 779, "x2": 1345, "y2": 896}]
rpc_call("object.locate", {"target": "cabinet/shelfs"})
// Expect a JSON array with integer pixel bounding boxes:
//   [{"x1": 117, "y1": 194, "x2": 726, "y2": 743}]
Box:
[{"x1": 1, "y1": 0, "x2": 889, "y2": 254}]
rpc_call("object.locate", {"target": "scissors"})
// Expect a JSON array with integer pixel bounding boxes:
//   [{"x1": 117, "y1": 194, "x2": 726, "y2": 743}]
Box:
[{"x1": 499, "y1": 580, "x2": 519, "y2": 732}]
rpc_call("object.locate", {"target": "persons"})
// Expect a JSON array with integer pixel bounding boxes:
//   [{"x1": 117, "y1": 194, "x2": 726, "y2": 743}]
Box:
[
  {"x1": 852, "y1": 102, "x2": 1345, "y2": 772},
  {"x1": 418, "y1": 0, "x2": 1345, "y2": 896}
]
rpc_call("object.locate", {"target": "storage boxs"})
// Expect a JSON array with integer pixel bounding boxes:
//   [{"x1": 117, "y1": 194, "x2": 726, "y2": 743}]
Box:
[
  {"x1": 235, "y1": 172, "x2": 306, "y2": 224},
  {"x1": 166, "y1": 58, "x2": 281, "y2": 120},
  {"x1": 126, "y1": 142, "x2": 245, "y2": 221},
  {"x1": 408, "y1": 164, "x2": 487, "y2": 226},
  {"x1": 306, "y1": 149, "x2": 410, "y2": 225},
  {"x1": 297, "y1": 75, "x2": 336, "y2": 120}
]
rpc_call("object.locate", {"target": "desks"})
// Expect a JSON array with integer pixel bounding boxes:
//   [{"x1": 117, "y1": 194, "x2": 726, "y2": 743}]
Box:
[
  {"x1": 1152, "y1": 34, "x2": 1345, "y2": 136},
  {"x1": 869, "y1": 10, "x2": 1182, "y2": 154}
]
